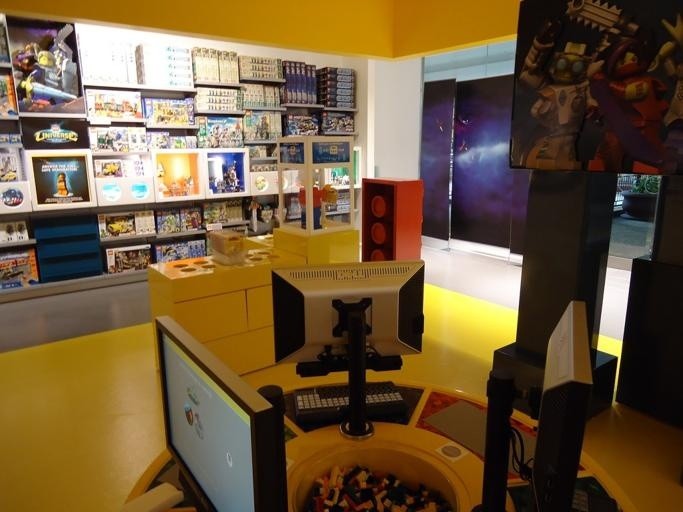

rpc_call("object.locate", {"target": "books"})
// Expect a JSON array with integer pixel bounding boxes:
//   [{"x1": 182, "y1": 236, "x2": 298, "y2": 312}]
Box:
[{"x1": 78, "y1": 33, "x2": 357, "y2": 114}]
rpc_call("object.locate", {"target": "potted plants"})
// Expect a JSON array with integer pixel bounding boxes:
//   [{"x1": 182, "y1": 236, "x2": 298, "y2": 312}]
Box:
[{"x1": 621, "y1": 176, "x2": 660, "y2": 221}]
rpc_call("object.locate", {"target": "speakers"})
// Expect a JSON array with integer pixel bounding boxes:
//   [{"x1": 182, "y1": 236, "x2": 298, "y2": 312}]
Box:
[{"x1": 360, "y1": 176, "x2": 425, "y2": 260}]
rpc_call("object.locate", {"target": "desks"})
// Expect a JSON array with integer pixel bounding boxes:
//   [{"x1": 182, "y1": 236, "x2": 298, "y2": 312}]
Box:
[{"x1": 0, "y1": 283, "x2": 682, "y2": 512}]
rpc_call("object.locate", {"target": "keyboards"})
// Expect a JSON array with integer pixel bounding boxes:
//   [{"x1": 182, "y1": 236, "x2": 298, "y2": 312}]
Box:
[{"x1": 291, "y1": 382, "x2": 405, "y2": 419}]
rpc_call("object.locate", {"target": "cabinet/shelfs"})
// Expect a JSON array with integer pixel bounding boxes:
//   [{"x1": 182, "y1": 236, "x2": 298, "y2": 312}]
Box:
[{"x1": 0, "y1": 11, "x2": 358, "y2": 353}]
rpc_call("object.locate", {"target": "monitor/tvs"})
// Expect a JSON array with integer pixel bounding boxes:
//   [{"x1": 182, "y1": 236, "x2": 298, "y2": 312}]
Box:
[
  {"x1": 270, "y1": 259, "x2": 426, "y2": 373},
  {"x1": 154, "y1": 315, "x2": 289, "y2": 507},
  {"x1": 529, "y1": 299, "x2": 594, "y2": 511}
]
